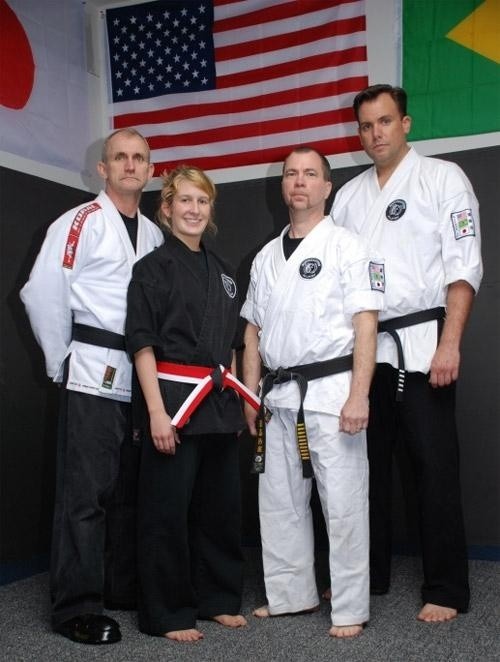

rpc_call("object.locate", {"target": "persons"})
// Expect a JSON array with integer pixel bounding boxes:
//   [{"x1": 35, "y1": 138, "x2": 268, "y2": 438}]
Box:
[
  {"x1": 239, "y1": 144, "x2": 392, "y2": 639},
  {"x1": 123, "y1": 167, "x2": 255, "y2": 644},
  {"x1": 18, "y1": 130, "x2": 167, "y2": 646},
  {"x1": 328, "y1": 83, "x2": 488, "y2": 625}
]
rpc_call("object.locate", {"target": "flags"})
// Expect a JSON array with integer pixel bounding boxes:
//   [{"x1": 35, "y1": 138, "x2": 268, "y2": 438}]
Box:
[
  {"x1": 1, "y1": 1, "x2": 93, "y2": 175},
  {"x1": 102, "y1": 1, "x2": 373, "y2": 177},
  {"x1": 393, "y1": 0, "x2": 500, "y2": 146}
]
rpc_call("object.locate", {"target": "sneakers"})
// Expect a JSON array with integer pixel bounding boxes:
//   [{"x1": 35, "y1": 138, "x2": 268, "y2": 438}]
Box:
[
  {"x1": 57, "y1": 612, "x2": 123, "y2": 646},
  {"x1": 102, "y1": 593, "x2": 143, "y2": 611}
]
